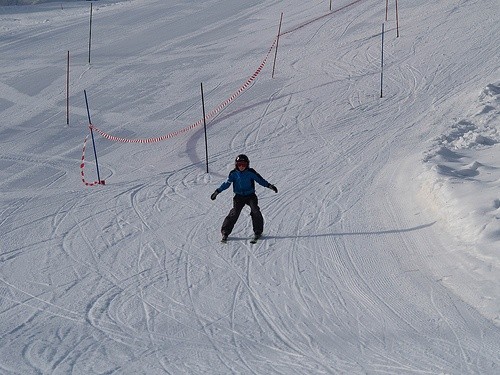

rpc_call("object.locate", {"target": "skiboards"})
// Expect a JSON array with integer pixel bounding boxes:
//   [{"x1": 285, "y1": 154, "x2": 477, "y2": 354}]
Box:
[
  {"x1": 221, "y1": 240, "x2": 226, "y2": 244},
  {"x1": 250, "y1": 235, "x2": 262, "y2": 244}
]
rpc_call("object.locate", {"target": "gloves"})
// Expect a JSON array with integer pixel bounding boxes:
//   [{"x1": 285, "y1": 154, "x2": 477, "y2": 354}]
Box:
[
  {"x1": 267, "y1": 184, "x2": 278, "y2": 193},
  {"x1": 211, "y1": 189, "x2": 220, "y2": 200}
]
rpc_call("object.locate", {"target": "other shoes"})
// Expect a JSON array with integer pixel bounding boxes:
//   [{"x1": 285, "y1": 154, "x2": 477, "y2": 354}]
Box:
[
  {"x1": 255, "y1": 235, "x2": 261, "y2": 239},
  {"x1": 222, "y1": 235, "x2": 228, "y2": 240}
]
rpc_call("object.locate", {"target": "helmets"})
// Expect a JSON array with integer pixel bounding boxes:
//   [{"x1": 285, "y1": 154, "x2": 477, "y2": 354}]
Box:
[{"x1": 235, "y1": 154, "x2": 249, "y2": 162}]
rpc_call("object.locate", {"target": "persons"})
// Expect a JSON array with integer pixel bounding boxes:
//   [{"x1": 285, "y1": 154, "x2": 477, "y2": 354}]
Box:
[{"x1": 211, "y1": 154, "x2": 278, "y2": 241}]
